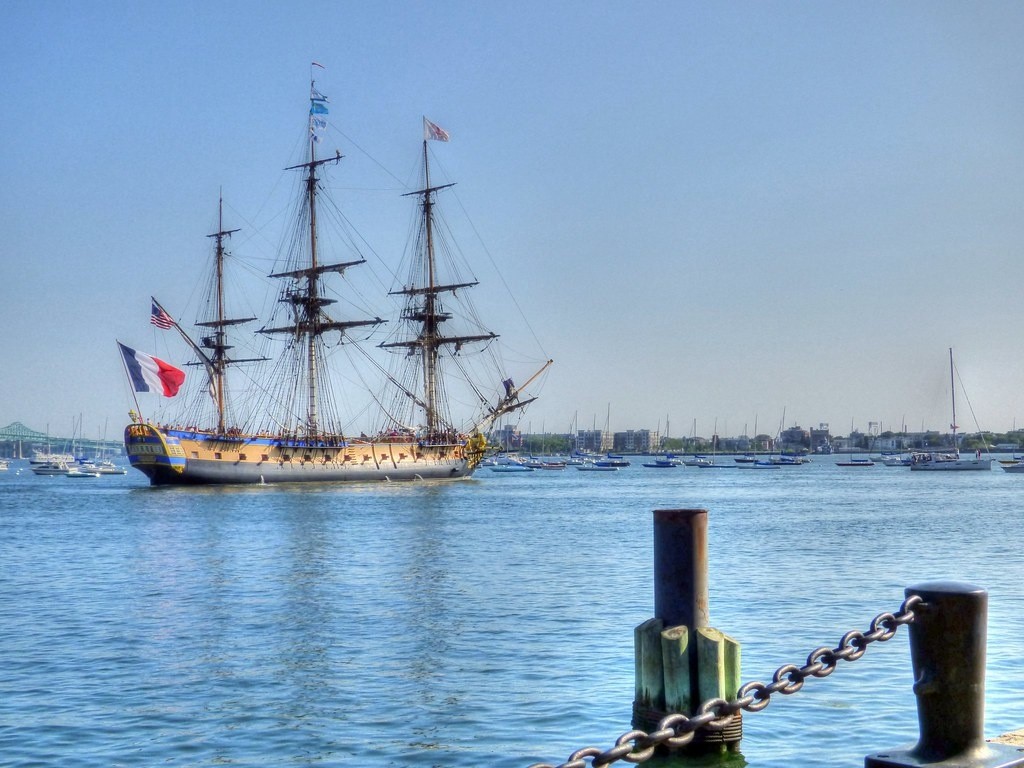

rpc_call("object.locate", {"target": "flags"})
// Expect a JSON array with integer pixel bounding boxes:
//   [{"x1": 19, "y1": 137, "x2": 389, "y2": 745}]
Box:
[
  {"x1": 150, "y1": 299, "x2": 175, "y2": 331},
  {"x1": 308, "y1": 80, "x2": 331, "y2": 144},
  {"x1": 312, "y1": 62, "x2": 325, "y2": 70},
  {"x1": 115, "y1": 338, "x2": 185, "y2": 396},
  {"x1": 423, "y1": 116, "x2": 449, "y2": 142}
]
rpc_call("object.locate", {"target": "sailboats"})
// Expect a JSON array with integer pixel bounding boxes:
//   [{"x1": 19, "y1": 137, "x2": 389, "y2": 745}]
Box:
[
  {"x1": 113, "y1": 60, "x2": 554, "y2": 488},
  {"x1": 29, "y1": 411, "x2": 127, "y2": 478},
  {"x1": 833, "y1": 347, "x2": 1024, "y2": 475},
  {"x1": 474, "y1": 400, "x2": 814, "y2": 472}
]
